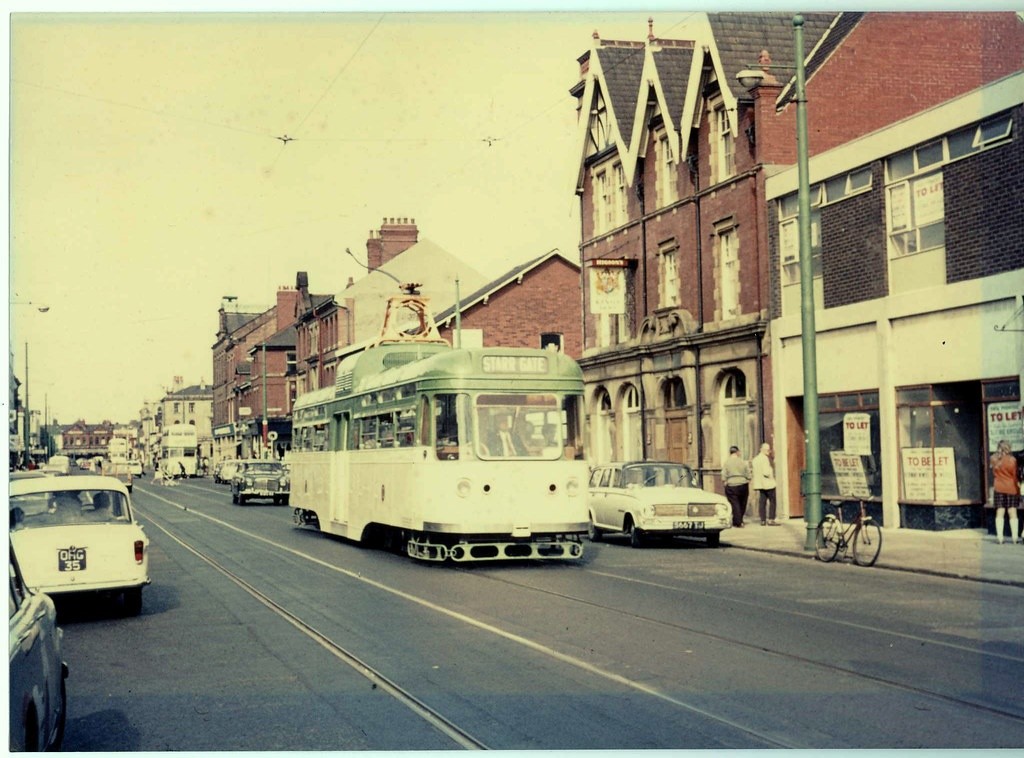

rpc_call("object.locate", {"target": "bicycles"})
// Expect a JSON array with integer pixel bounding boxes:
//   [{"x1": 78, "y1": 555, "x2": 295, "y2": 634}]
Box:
[{"x1": 814, "y1": 493, "x2": 882, "y2": 567}]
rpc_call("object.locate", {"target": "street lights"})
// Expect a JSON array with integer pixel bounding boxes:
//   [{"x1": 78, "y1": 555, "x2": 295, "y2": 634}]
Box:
[{"x1": 735, "y1": 11, "x2": 827, "y2": 552}]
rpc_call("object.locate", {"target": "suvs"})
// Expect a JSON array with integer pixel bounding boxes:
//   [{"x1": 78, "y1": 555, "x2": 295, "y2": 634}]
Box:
[{"x1": 233, "y1": 458, "x2": 291, "y2": 506}]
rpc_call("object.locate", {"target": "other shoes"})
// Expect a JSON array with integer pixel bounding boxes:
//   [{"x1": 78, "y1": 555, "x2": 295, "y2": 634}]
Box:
[
  {"x1": 761, "y1": 521, "x2": 766, "y2": 526},
  {"x1": 737, "y1": 523, "x2": 744, "y2": 528},
  {"x1": 768, "y1": 520, "x2": 781, "y2": 526}
]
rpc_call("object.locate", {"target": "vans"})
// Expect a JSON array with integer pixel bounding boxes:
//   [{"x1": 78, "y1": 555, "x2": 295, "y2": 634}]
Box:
[{"x1": 214, "y1": 459, "x2": 238, "y2": 485}]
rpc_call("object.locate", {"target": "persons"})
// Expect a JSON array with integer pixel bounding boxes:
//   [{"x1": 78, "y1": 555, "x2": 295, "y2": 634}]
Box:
[
  {"x1": 751, "y1": 443, "x2": 781, "y2": 525},
  {"x1": 10, "y1": 460, "x2": 40, "y2": 472},
  {"x1": 10, "y1": 490, "x2": 109, "y2": 529},
  {"x1": 202, "y1": 456, "x2": 209, "y2": 477},
  {"x1": 150, "y1": 457, "x2": 164, "y2": 486},
  {"x1": 990, "y1": 440, "x2": 1020, "y2": 544},
  {"x1": 365, "y1": 411, "x2": 558, "y2": 456},
  {"x1": 722, "y1": 446, "x2": 752, "y2": 527}
]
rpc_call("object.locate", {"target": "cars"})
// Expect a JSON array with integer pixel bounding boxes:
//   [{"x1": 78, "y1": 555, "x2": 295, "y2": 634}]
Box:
[
  {"x1": 10, "y1": 472, "x2": 151, "y2": 619},
  {"x1": 9, "y1": 530, "x2": 69, "y2": 753},
  {"x1": 10, "y1": 446, "x2": 145, "y2": 518},
  {"x1": 588, "y1": 459, "x2": 733, "y2": 548}
]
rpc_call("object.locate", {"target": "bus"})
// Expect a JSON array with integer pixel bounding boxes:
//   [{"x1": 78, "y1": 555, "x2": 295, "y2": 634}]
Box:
[
  {"x1": 158, "y1": 424, "x2": 200, "y2": 479},
  {"x1": 288, "y1": 336, "x2": 590, "y2": 564}
]
